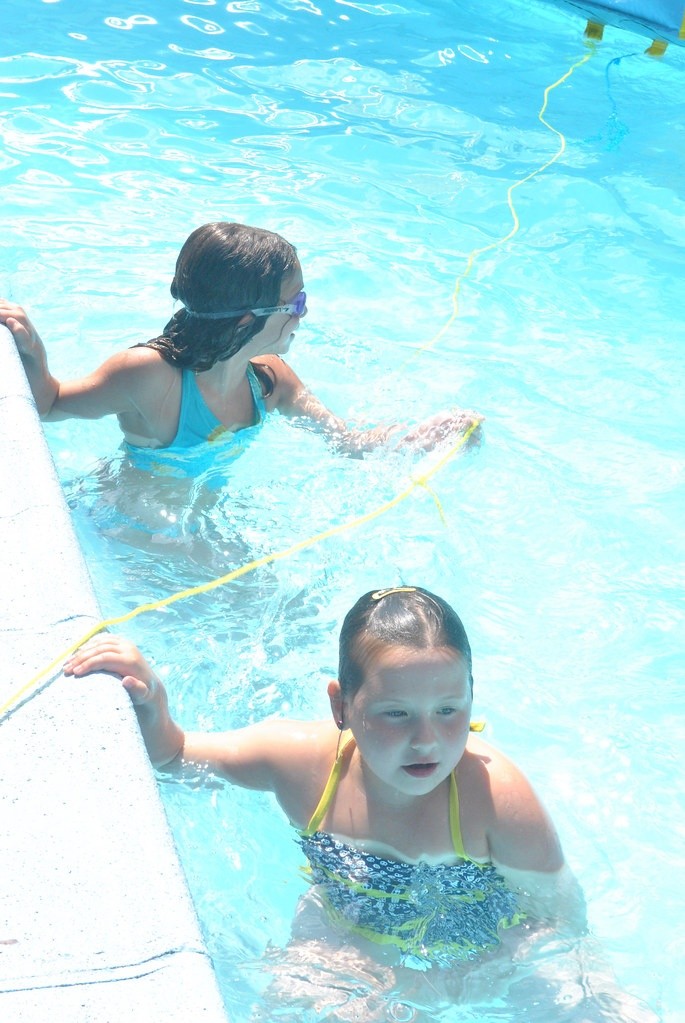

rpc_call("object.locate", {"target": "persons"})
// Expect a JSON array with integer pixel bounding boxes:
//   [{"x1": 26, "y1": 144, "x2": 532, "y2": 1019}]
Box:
[
  {"x1": 1, "y1": 222, "x2": 478, "y2": 463},
  {"x1": 63, "y1": 585, "x2": 565, "y2": 877}
]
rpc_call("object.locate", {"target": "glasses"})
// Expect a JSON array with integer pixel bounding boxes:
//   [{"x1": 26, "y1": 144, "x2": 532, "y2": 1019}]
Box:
[{"x1": 289, "y1": 291, "x2": 306, "y2": 318}]
